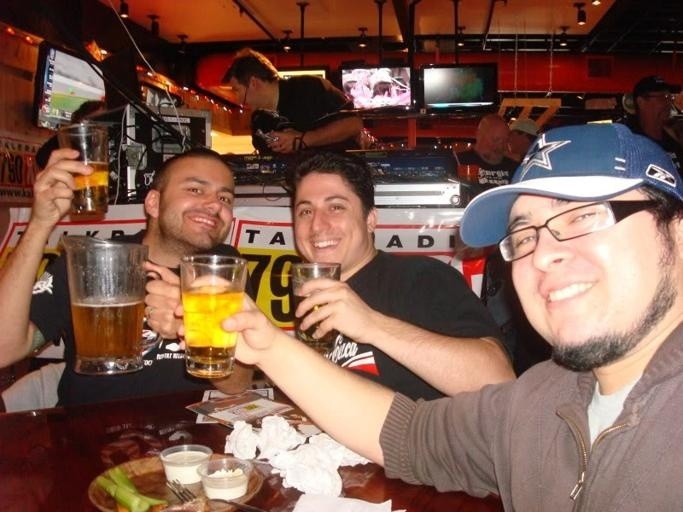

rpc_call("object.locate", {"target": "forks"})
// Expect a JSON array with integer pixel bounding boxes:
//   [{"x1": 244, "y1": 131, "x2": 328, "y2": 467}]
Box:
[{"x1": 165, "y1": 478, "x2": 268, "y2": 512}]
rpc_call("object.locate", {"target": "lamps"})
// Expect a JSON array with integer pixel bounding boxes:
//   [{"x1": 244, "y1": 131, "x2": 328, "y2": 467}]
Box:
[
  {"x1": 457, "y1": 27, "x2": 467, "y2": 46},
  {"x1": 560, "y1": 24, "x2": 571, "y2": 46},
  {"x1": 574, "y1": 1, "x2": 587, "y2": 25},
  {"x1": 281, "y1": 30, "x2": 293, "y2": 50},
  {"x1": 357, "y1": 27, "x2": 367, "y2": 48},
  {"x1": 119, "y1": 0, "x2": 128, "y2": 19}
]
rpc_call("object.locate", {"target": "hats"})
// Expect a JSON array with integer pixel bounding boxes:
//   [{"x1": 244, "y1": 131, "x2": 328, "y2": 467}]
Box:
[
  {"x1": 457, "y1": 126, "x2": 678, "y2": 255},
  {"x1": 632, "y1": 75, "x2": 683, "y2": 96},
  {"x1": 509, "y1": 118, "x2": 540, "y2": 137}
]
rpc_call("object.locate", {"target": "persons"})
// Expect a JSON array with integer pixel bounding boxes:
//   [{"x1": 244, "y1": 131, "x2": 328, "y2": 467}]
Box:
[
  {"x1": 173, "y1": 120, "x2": 683, "y2": 512},
  {"x1": 343, "y1": 76, "x2": 367, "y2": 109},
  {"x1": 454, "y1": 116, "x2": 520, "y2": 195},
  {"x1": 0, "y1": 148, "x2": 254, "y2": 412},
  {"x1": 508, "y1": 117, "x2": 539, "y2": 161},
  {"x1": 615, "y1": 75, "x2": 683, "y2": 176},
  {"x1": 221, "y1": 48, "x2": 363, "y2": 156},
  {"x1": 391, "y1": 77, "x2": 411, "y2": 105},
  {"x1": 35, "y1": 99, "x2": 108, "y2": 168},
  {"x1": 282, "y1": 151, "x2": 516, "y2": 397},
  {"x1": 369, "y1": 81, "x2": 395, "y2": 108}
]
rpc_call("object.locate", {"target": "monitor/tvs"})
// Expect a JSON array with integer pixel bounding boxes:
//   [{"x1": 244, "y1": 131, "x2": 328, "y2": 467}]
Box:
[
  {"x1": 276, "y1": 66, "x2": 329, "y2": 83},
  {"x1": 421, "y1": 62, "x2": 499, "y2": 113},
  {"x1": 139, "y1": 79, "x2": 181, "y2": 110},
  {"x1": 34, "y1": 41, "x2": 106, "y2": 131},
  {"x1": 340, "y1": 65, "x2": 413, "y2": 113}
]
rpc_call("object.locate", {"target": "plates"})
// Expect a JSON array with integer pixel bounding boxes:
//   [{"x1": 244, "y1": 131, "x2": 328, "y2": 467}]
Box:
[{"x1": 88, "y1": 452, "x2": 263, "y2": 512}]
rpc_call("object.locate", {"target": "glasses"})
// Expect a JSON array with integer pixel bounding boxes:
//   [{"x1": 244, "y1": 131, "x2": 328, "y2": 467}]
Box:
[
  {"x1": 646, "y1": 91, "x2": 676, "y2": 102},
  {"x1": 494, "y1": 196, "x2": 666, "y2": 261}
]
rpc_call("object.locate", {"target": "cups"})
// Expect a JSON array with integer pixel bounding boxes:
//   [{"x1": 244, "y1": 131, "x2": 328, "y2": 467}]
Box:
[
  {"x1": 456, "y1": 164, "x2": 480, "y2": 203},
  {"x1": 58, "y1": 121, "x2": 110, "y2": 215},
  {"x1": 60, "y1": 233, "x2": 165, "y2": 377},
  {"x1": 179, "y1": 253, "x2": 248, "y2": 379},
  {"x1": 290, "y1": 263, "x2": 340, "y2": 354}
]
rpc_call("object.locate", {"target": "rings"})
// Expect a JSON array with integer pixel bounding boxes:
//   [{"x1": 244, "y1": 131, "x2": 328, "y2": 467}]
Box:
[{"x1": 147, "y1": 304, "x2": 157, "y2": 318}]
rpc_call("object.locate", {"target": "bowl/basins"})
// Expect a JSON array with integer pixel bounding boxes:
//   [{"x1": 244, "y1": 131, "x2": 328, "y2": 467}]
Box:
[
  {"x1": 196, "y1": 458, "x2": 250, "y2": 500},
  {"x1": 160, "y1": 444, "x2": 212, "y2": 485}
]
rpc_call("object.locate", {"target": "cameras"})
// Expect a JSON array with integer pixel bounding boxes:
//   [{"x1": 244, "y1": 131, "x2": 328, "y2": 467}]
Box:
[{"x1": 250, "y1": 109, "x2": 291, "y2": 155}]
